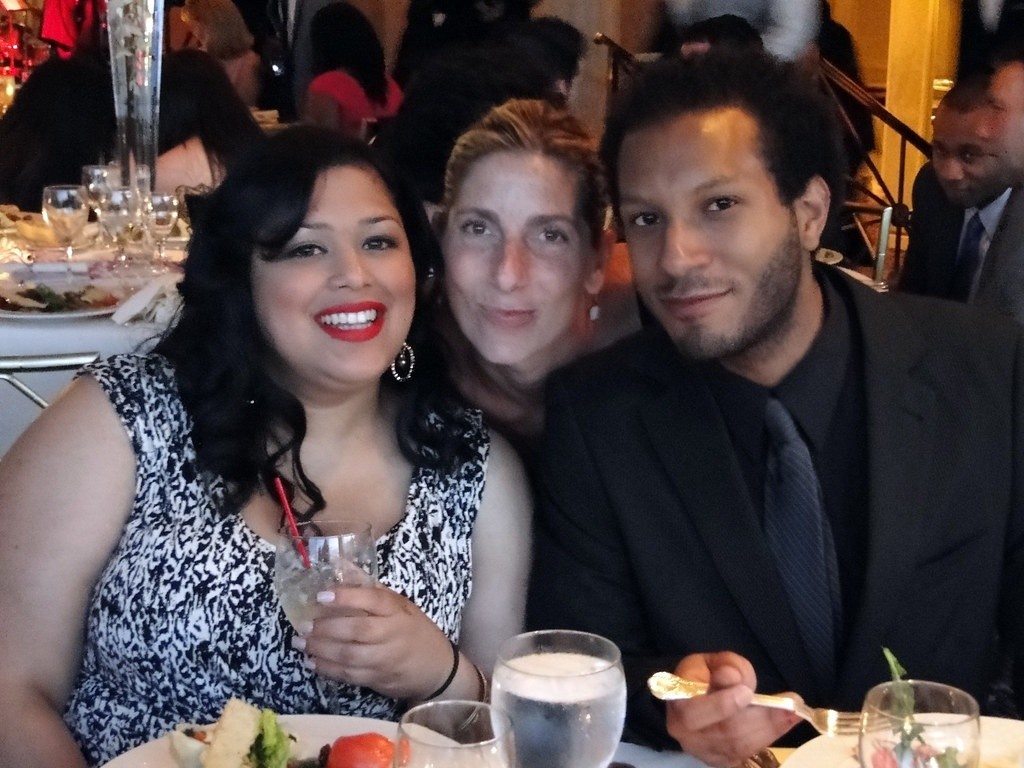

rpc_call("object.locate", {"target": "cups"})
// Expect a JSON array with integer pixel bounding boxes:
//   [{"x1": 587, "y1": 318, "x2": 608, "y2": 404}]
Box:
[
  {"x1": 858, "y1": 680, "x2": 980, "y2": 768},
  {"x1": 490, "y1": 630, "x2": 628, "y2": 768},
  {"x1": 391, "y1": 700, "x2": 516, "y2": 768}
]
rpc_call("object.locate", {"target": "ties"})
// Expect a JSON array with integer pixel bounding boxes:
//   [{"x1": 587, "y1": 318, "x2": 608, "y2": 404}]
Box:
[
  {"x1": 762, "y1": 396, "x2": 845, "y2": 712},
  {"x1": 950, "y1": 212, "x2": 984, "y2": 304}
]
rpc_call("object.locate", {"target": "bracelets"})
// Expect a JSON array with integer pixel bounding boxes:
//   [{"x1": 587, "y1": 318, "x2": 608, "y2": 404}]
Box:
[
  {"x1": 420, "y1": 639, "x2": 460, "y2": 703},
  {"x1": 456, "y1": 664, "x2": 492, "y2": 733}
]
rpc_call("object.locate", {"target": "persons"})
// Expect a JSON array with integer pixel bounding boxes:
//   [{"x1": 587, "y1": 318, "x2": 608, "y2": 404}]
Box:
[
  {"x1": 430, "y1": 99, "x2": 645, "y2": 440},
  {"x1": 523, "y1": 46, "x2": 1024, "y2": 768},
  {"x1": 0, "y1": 126, "x2": 532, "y2": 768},
  {"x1": 0, "y1": 0, "x2": 876, "y2": 222},
  {"x1": 889, "y1": 56, "x2": 1024, "y2": 323}
]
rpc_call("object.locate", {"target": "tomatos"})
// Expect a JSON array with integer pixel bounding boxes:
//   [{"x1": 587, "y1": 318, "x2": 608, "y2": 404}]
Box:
[{"x1": 327, "y1": 733, "x2": 394, "y2": 768}]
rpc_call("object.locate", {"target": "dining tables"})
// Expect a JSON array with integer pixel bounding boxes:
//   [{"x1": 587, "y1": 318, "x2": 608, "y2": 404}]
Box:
[{"x1": 0, "y1": 204, "x2": 189, "y2": 454}]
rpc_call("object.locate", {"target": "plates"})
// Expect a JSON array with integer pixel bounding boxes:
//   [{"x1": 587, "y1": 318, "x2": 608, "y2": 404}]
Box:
[
  {"x1": 0, "y1": 212, "x2": 191, "y2": 266},
  {"x1": 815, "y1": 248, "x2": 844, "y2": 265},
  {"x1": 0, "y1": 261, "x2": 183, "y2": 319},
  {"x1": 98, "y1": 714, "x2": 488, "y2": 768},
  {"x1": 778, "y1": 712, "x2": 1024, "y2": 766}
]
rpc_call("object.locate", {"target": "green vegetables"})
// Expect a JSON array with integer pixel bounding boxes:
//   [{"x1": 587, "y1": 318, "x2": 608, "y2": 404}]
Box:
[
  {"x1": 880, "y1": 645, "x2": 963, "y2": 768},
  {"x1": 0, "y1": 282, "x2": 96, "y2": 313}
]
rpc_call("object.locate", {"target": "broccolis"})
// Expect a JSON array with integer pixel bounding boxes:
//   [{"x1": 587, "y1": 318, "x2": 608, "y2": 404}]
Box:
[{"x1": 244, "y1": 706, "x2": 294, "y2": 768}]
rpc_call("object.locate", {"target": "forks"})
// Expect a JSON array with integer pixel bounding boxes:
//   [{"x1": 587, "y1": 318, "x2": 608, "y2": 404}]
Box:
[{"x1": 647, "y1": 671, "x2": 905, "y2": 738}]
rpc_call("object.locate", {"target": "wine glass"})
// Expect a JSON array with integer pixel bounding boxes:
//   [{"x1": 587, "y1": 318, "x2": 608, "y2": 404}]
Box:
[
  {"x1": 43, "y1": 162, "x2": 179, "y2": 285},
  {"x1": 274, "y1": 519, "x2": 379, "y2": 714}
]
